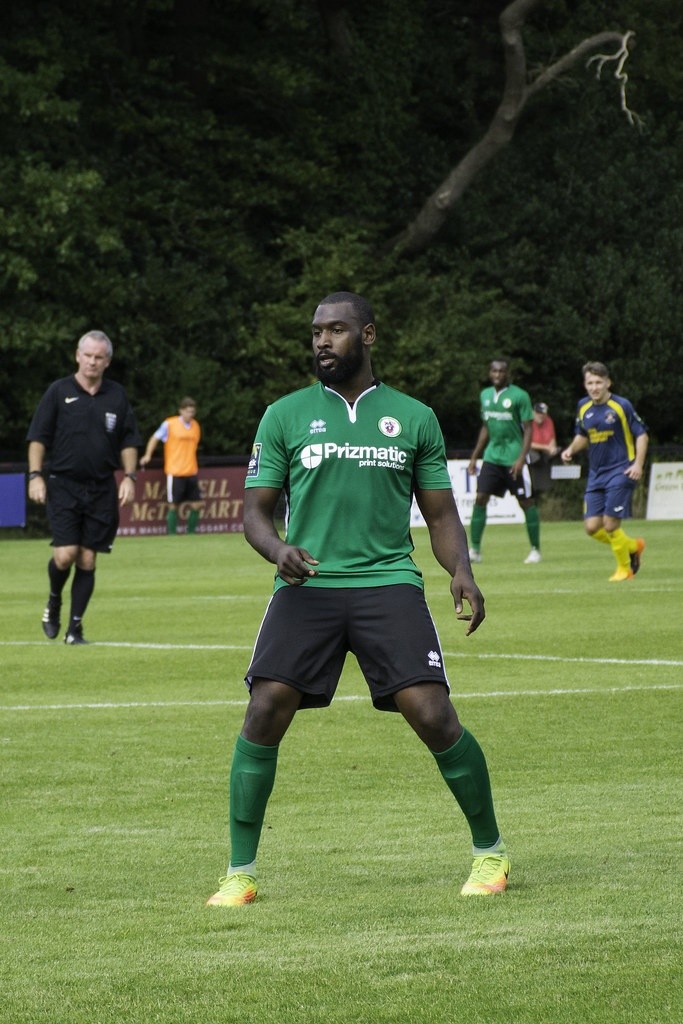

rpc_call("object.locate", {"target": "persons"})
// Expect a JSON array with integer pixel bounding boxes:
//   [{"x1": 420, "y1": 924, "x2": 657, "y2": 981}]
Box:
[
  {"x1": 202, "y1": 292, "x2": 513, "y2": 907},
  {"x1": 25, "y1": 329, "x2": 140, "y2": 647},
  {"x1": 466, "y1": 358, "x2": 542, "y2": 565},
  {"x1": 529, "y1": 403, "x2": 559, "y2": 500},
  {"x1": 563, "y1": 361, "x2": 649, "y2": 582},
  {"x1": 140, "y1": 396, "x2": 202, "y2": 537}
]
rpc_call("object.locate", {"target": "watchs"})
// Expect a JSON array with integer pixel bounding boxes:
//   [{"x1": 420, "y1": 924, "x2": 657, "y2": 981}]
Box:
[
  {"x1": 27, "y1": 471, "x2": 43, "y2": 480},
  {"x1": 123, "y1": 472, "x2": 138, "y2": 483}
]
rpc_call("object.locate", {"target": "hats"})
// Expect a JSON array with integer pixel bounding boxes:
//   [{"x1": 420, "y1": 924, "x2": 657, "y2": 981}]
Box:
[{"x1": 534, "y1": 402, "x2": 548, "y2": 415}]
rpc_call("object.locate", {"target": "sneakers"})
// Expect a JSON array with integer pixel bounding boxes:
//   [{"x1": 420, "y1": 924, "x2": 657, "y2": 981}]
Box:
[
  {"x1": 606, "y1": 568, "x2": 633, "y2": 581},
  {"x1": 206, "y1": 875, "x2": 258, "y2": 908},
  {"x1": 42, "y1": 596, "x2": 62, "y2": 639},
  {"x1": 65, "y1": 628, "x2": 87, "y2": 645},
  {"x1": 469, "y1": 547, "x2": 482, "y2": 563},
  {"x1": 461, "y1": 849, "x2": 511, "y2": 897},
  {"x1": 524, "y1": 549, "x2": 542, "y2": 565},
  {"x1": 629, "y1": 538, "x2": 645, "y2": 574}
]
